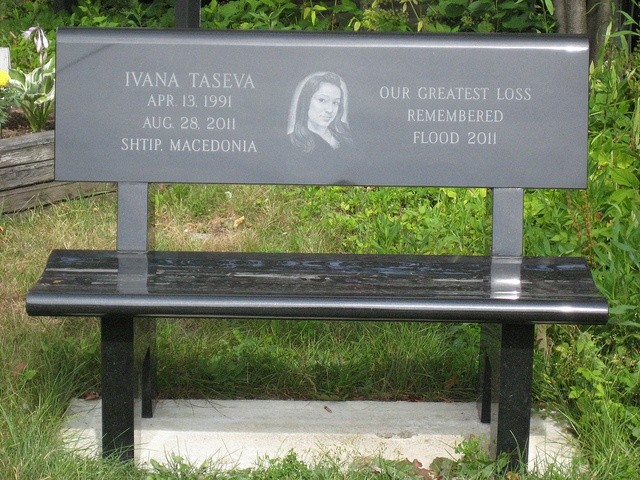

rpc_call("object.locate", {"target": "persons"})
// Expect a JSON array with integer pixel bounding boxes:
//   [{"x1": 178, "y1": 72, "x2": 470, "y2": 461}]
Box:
[{"x1": 287, "y1": 71, "x2": 354, "y2": 155}]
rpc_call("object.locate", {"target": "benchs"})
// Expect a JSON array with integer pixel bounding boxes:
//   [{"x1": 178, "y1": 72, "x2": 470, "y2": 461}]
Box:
[{"x1": 27, "y1": 24, "x2": 611, "y2": 474}]
